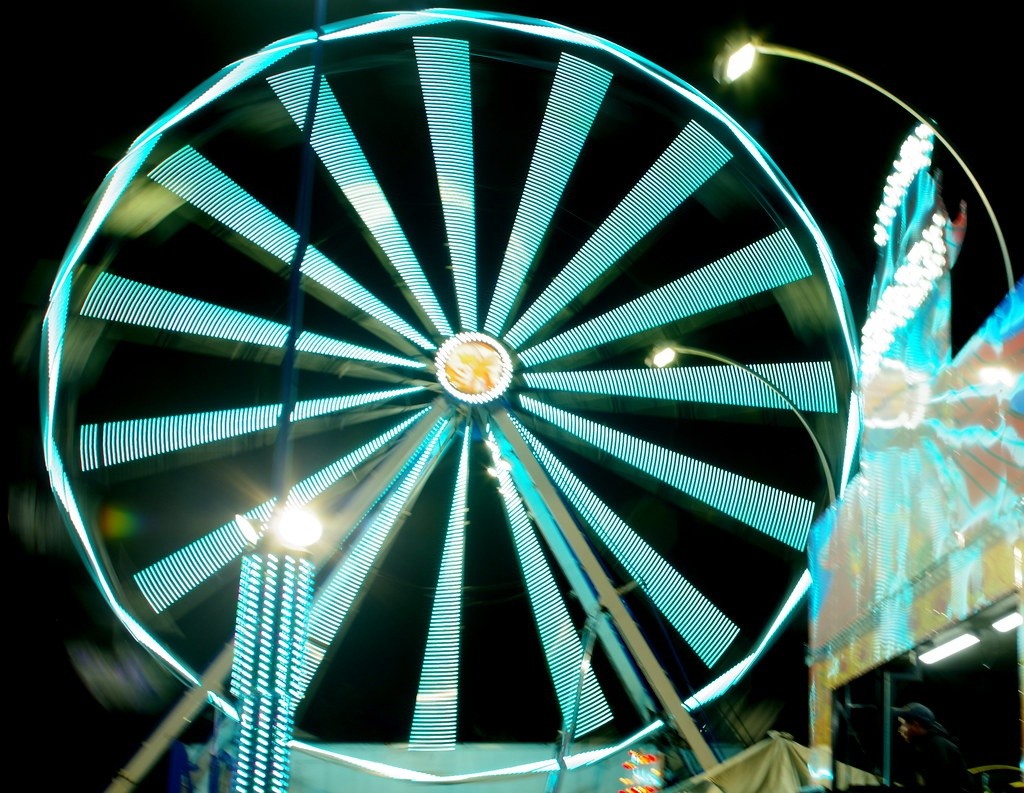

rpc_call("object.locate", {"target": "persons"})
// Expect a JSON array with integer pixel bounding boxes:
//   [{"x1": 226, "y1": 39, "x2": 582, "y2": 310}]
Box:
[{"x1": 897, "y1": 703, "x2": 972, "y2": 793}]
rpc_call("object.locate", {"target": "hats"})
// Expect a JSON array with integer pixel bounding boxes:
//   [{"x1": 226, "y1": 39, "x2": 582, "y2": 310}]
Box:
[{"x1": 891, "y1": 703, "x2": 934, "y2": 728}]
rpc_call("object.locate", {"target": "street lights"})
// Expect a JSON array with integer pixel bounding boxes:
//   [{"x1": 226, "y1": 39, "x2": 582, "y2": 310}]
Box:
[
  {"x1": 644, "y1": 342, "x2": 837, "y2": 504},
  {"x1": 713, "y1": 32, "x2": 1015, "y2": 291}
]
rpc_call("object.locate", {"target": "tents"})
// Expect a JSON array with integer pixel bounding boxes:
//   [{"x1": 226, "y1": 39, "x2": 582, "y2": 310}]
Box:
[{"x1": 656, "y1": 730, "x2": 904, "y2": 793}]
